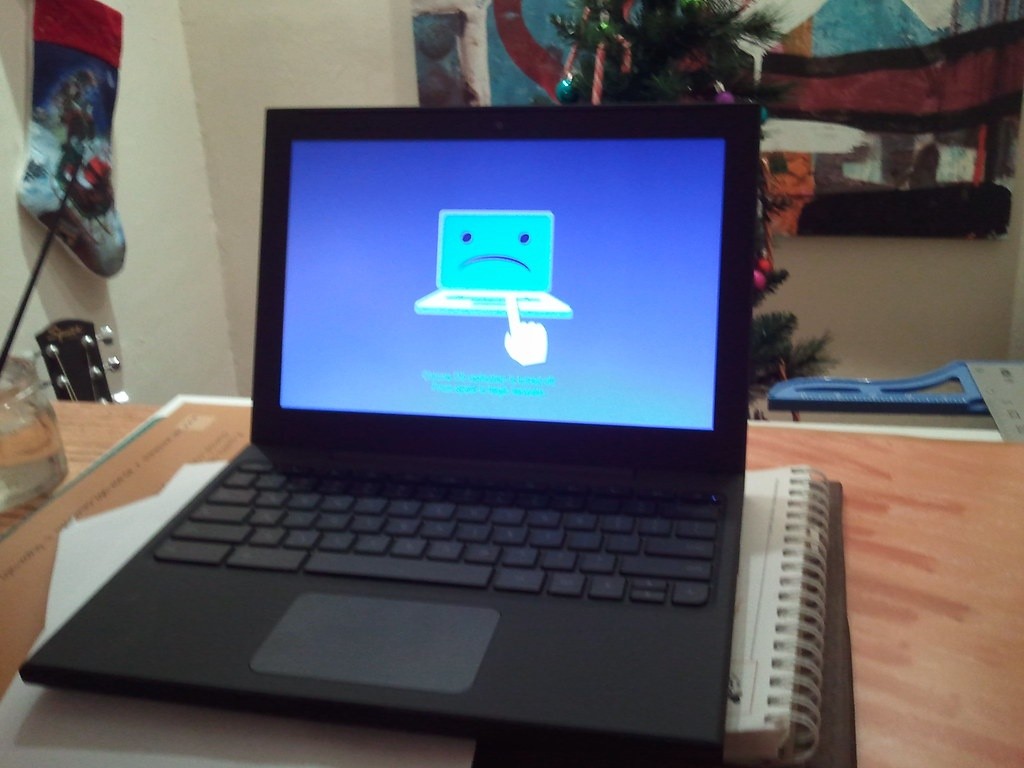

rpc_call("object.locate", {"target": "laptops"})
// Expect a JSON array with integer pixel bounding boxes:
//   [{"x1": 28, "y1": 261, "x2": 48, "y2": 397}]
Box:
[{"x1": 17, "y1": 102, "x2": 760, "y2": 763}]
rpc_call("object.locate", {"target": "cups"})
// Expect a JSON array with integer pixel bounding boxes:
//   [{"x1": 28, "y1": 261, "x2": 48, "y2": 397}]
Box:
[{"x1": 0, "y1": 358, "x2": 69, "y2": 512}]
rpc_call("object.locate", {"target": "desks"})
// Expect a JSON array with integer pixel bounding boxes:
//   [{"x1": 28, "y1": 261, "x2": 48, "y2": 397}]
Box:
[{"x1": 1, "y1": 394, "x2": 1023, "y2": 767}]
